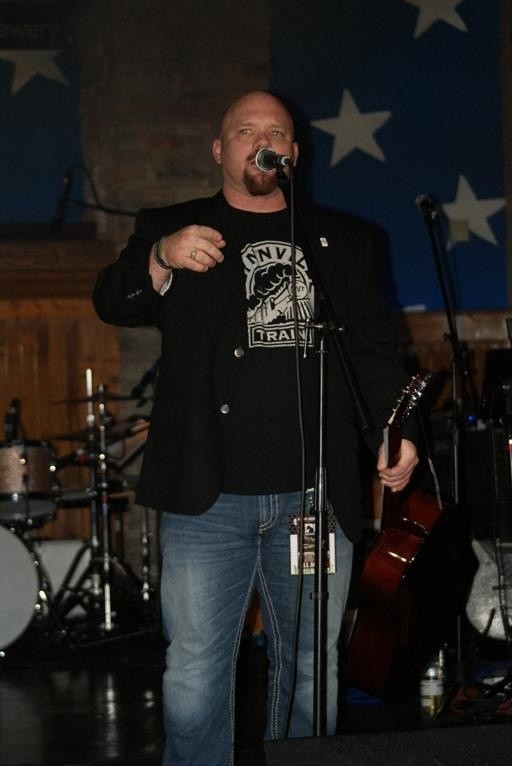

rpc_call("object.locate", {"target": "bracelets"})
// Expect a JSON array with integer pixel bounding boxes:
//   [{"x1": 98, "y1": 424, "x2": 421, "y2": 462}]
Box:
[{"x1": 153, "y1": 234, "x2": 175, "y2": 269}]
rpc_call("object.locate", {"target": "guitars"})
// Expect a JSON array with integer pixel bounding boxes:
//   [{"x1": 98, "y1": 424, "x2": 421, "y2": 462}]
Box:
[{"x1": 363, "y1": 370, "x2": 441, "y2": 600}]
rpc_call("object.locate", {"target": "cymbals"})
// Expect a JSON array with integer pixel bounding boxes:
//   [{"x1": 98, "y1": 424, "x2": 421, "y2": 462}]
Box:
[{"x1": 50, "y1": 392, "x2": 140, "y2": 402}]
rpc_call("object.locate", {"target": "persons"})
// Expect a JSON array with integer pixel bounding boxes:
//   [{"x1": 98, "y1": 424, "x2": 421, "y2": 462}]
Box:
[{"x1": 91, "y1": 85, "x2": 422, "y2": 766}]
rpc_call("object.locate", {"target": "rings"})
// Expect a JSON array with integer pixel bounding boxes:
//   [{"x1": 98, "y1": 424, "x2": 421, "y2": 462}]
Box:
[{"x1": 191, "y1": 247, "x2": 198, "y2": 258}]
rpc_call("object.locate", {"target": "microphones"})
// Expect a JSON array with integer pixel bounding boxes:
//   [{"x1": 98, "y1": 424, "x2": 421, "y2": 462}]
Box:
[
  {"x1": 413, "y1": 189, "x2": 439, "y2": 219},
  {"x1": 254, "y1": 147, "x2": 292, "y2": 175}
]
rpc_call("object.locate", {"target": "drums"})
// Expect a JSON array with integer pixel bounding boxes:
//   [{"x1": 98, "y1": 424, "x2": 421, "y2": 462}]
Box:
[
  {"x1": 30, "y1": 540, "x2": 98, "y2": 621},
  {"x1": 2, "y1": 525, "x2": 49, "y2": 651},
  {"x1": 0, "y1": 443, "x2": 59, "y2": 520}
]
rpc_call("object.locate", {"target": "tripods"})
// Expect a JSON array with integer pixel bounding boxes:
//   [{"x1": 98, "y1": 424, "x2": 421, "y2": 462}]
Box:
[{"x1": 61, "y1": 455, "x2": 147, "y2": 632}]
